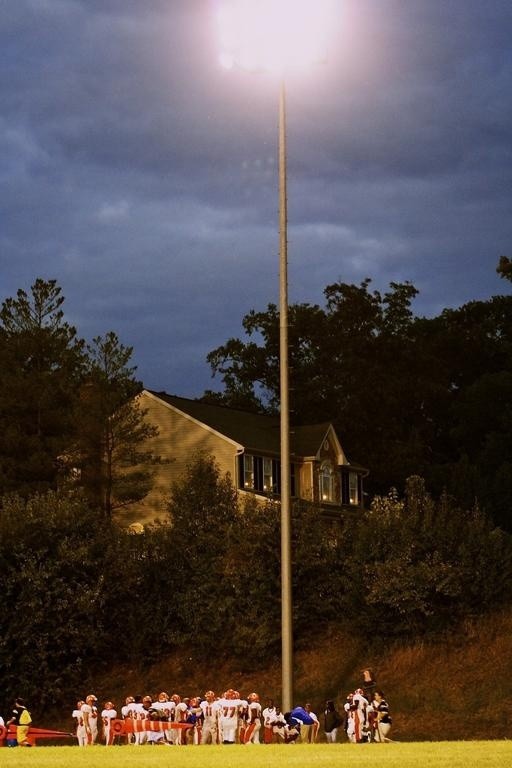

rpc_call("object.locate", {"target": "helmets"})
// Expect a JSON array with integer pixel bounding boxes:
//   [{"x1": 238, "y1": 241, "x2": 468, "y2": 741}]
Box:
[
  {"x1": 76, "y1": 689, "x2": 259, "y2": 709},
  {"x1": 346, "y1": 687, "x2": 364, "y2": 700}
]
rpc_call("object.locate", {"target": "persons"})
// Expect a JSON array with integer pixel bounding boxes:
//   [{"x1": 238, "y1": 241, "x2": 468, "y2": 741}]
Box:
[
  {"x1": 73, "y1": 688, "x2": 320, "y2": 744},
  {"x1": 6, "y1": 698, "x2": 32, "y2": 747},
  {"x1": 323, "y1": 700, "x2": 343, "y2": 744},
  {"x1": 344, "y1": 688, "x2": 390, "y2": 743}
]
